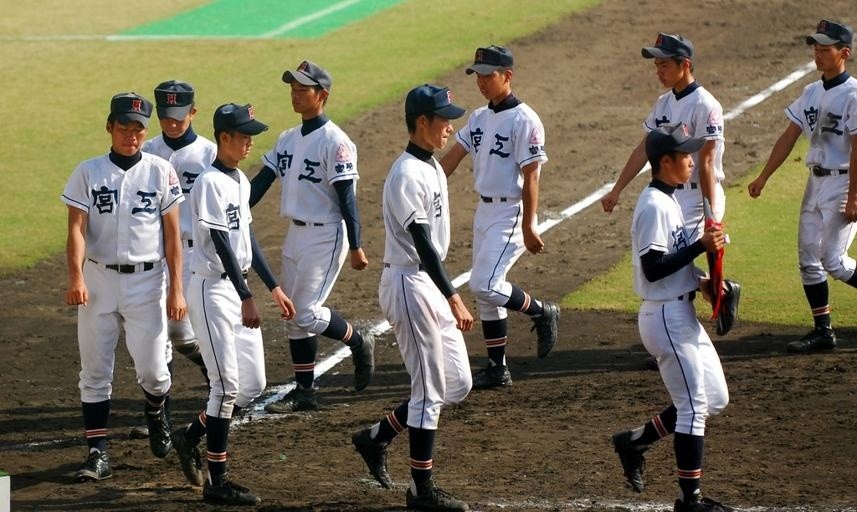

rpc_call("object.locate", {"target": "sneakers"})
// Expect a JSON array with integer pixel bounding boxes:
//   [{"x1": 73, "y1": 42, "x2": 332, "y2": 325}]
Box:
[
  {"x1": 406, "y1": 487, "x2": 470, "y2": 511},
  {"x1": 203, "y1": 473, "x2": 262, "y2": 504},
  {"x1": 264, "y1": 386, "x2": 319, "y2": 413},
  {"x1": 170, "y1": 428, "x2": 204, "y2": 486},
  {"x1": 471, "y1": 362, "x2": 514, "y2": 390},
  {"x1": 531, "y1": 299, "x2": 560, "y2": 361},
  {"x1": 75, "y1": 450, "x2": 113, "y2": 481},
  {"x1": 144, "y1": 401, "x2": 172, "y2": 457},
  {"x1": 613, "y1": 431, "x2": 644, "y2": 494},
  {"x1": 673, "y1": 498, "x2": 737, "y2": 511},
  {"x1": 787, "y1": 326, "x2": 836, "y2": 353},
  {"x1": 350, "y1": 335, "x2": 376, "y2": 392},
  {"x1": 350, "y1": 427, "x2": 394, "y2": 492},
  {"x1": 712, "y1": 280, "x2": 740, "y2": 335}
]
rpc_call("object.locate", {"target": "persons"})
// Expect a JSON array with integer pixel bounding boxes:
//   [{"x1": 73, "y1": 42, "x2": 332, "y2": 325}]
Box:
[
  {"x1": 249, "y1": 58, "x2": 376, "y2": 413},
  {"x1": 168, "y1": 102, "x2": 296, "y2": 506},
  {"x1": 612, "y1": 122, "x2": 736, "y2": 512},
  {"x1": 601, "y1": 31, "x2": 742, "y2": 336},
  {"x1": 131, "y1": 80, "x2": 218, "y2": 440},
  {"x1": 352, "y1": 83, "x2": 473, "y2": 511},
  {"x1": 747, "y1": 19, "x2": 857, "y2": 354},
  {"x1": 58, "y1": 91, "x2": 188, "y2": 482},
  {"x1": 439, "y1": 44, "x2": 561, "y2": 389}
]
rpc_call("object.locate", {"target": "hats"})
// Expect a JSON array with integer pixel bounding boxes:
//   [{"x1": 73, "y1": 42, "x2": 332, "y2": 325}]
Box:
[
  {"x1": 405, "y1": 85, "x2": 466, "y2": 119},
  {"x1": 466, "y1": 45, "x2": 514, "y2": 76},
  {"x1": 806, "y1": 19, "x2": 852, "y2": 46},
  {"x1": 111, "y1": 92, "x2": 153, "y2": 129},
  {"x1": 641, "y1": 33, "x2": 694, "y2": 60},
  {"x1": 214, "y1": 103, "x2": 269, "y2": 135},
  {"x1": 282, "y1": 61, "x2": 331, "y2": 93},
  {"x1": 154, "y1": 80, "x2": 194, "y2": 122},
  {"x1": 645, "y1": 122, "x2": 706, "y2": 158}
]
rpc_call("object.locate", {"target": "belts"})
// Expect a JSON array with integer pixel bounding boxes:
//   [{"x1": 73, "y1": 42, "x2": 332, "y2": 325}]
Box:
[
  {"x1": 89, "y1": 258, "x2": 154, "y2": 273},
  {"x1": 808, "y1": 165, "x2": 847, "y2": 176},
  {"x1": 220, "y1": 271, "x2": 248, "y2": 282},
  {"x1": 676, "y1": 183, "x2": 698, "y2": 190},
  {"x1": 480, "y1": 196, "x2": 507, "y2": 203},
  {"x1": 187, "y1": 239, "x2": 194, "y2": 247},
  {"x1": 292, "y1": 219, "x2": 324, "y2": 227},
  {"x1": 678, "y1": 291, "x2": 696, "y2": 302},
  {"x1": 383, "y1": 263, "x2": 426, "y2": 271}
]
